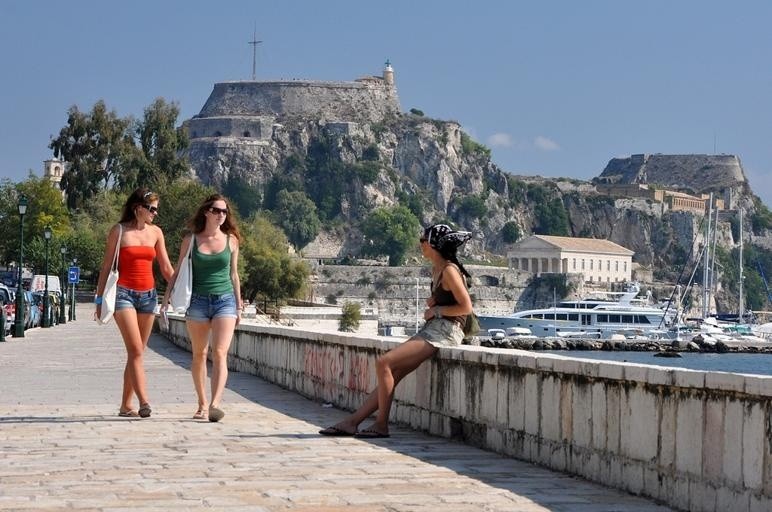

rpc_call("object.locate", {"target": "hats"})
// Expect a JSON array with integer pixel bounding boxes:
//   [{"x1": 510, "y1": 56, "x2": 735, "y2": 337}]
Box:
[{"x1": 425, "y1": 224, "x2": 473, "y2": 249}]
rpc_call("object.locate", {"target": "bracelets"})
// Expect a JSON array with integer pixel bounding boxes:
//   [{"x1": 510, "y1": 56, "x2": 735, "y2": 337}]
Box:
[
  {"x1": 95, "y1": 296, "x2": 102, "y2": 304},
  {"x1": 236, "y1": 306, "x2": 243, "y2": 310},
  {"x1": 432, "y1": 306, "x2": 443, "y2": 319}
]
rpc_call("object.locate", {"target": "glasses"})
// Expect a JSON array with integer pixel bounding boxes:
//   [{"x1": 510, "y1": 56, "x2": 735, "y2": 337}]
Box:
[
  {"x1": 142, "y1": 204, "x2": 158, "y2": 213},
  {"x1": 208, "y1": 206, "x2": 229, "y2": 215}
]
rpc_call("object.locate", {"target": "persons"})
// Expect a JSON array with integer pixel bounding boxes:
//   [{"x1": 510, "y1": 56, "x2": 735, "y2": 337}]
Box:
[
  {"x1": 317, "y1": 223, "x2": 473, "y2": 440},
  {"x1": 95, "y1": 187, "x2": 175, "y2": 418},
  {"x1": 159, "y1": 193, "x2": 244, "y2": 423}
]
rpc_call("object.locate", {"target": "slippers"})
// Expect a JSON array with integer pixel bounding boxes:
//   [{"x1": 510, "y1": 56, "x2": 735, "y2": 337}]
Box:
[
  {"x1": 193, "y1": 405, "x2": 209, "y2": 421},
  {"x1": 139, "y1": 403, "x2": 153, "y2": 418},
  {"x1": 319, "y1": 426, "x2": 358, "y2": 436},
  {"x1": 209, "y1": 406, "x2": 224, "y2": 422},
  {"x1": 355, "y1": 429, "x2": 391, "y2": 438},
  {"x1": 119, "y1": 404, "x2": 139, "y2": 417}
]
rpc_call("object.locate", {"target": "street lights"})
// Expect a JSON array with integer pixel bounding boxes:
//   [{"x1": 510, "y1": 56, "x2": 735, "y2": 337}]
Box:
[
  {"x1": 58, "y1": 242, "x2": 67, "y2": 323},
  {"x1": 414, "y1": 277, "x2": 419, "y2": 333},
  {"x1": 68, "y1": 256, "x2": 78, "y2": 321},
  {"x1": 42, "y1": 223, "x2": 52, "y2": 326},
  {"x1": 13, "y1": 193, "x2": 29, "y2": 338}
]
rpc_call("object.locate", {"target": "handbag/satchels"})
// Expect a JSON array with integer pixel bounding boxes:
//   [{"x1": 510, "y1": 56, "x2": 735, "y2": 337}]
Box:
[
  {"x1": 463, "y1": 312, "x2": 480, "y2": 336},
  {"x1": 168, "y1": 257, "x2": 192, "y2": 314},
  {"x1": 94, "y1": 269, "x2": 119, "y2": 325}
]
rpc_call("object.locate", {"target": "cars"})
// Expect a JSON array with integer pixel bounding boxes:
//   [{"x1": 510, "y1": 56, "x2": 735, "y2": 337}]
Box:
[{"x1": 0, "y1": 270, "x2": 67, "y2": 336}]
